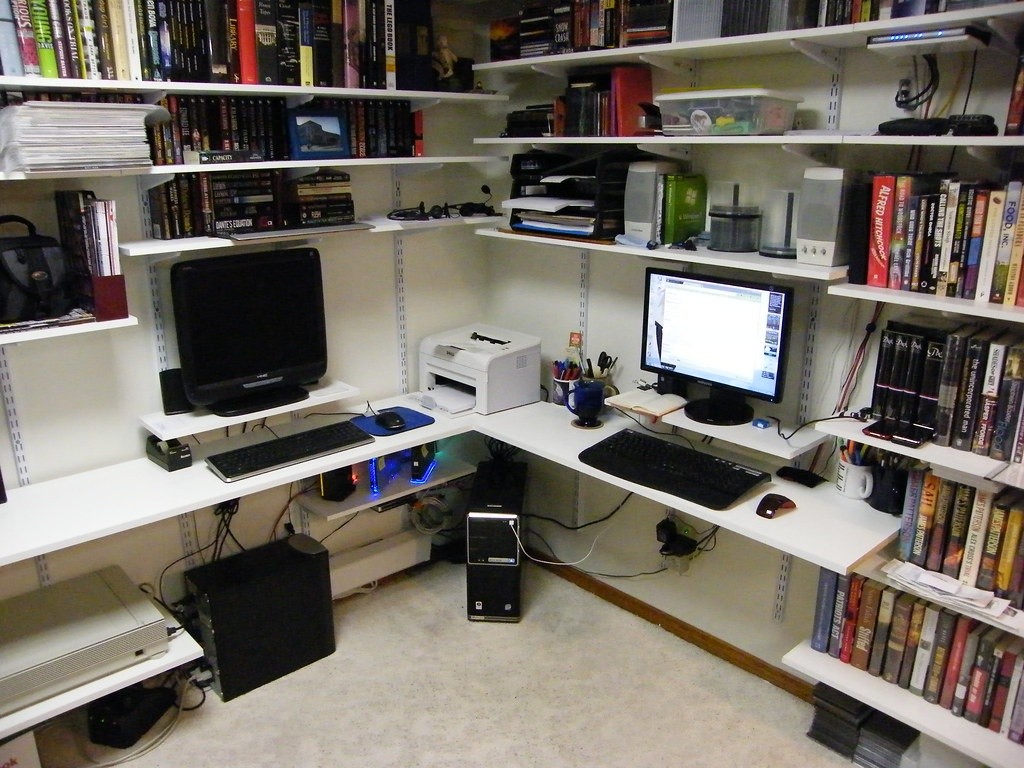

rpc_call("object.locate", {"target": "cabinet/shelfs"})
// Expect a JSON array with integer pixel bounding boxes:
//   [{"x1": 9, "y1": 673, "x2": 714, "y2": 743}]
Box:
[{"x1": 0, "y1": 1, "x2": 1024, "y2": 768}]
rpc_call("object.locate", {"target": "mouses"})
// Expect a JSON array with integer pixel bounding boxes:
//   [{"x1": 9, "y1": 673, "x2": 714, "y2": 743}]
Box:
[{"x1": 375, "y1": 412, "x2": 406, "y2": 429}]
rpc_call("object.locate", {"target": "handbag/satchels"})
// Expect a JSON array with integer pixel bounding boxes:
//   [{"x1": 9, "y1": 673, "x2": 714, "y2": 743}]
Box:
[{"x1": 0, "y1": 214, "x2": 77, "y2": 324}]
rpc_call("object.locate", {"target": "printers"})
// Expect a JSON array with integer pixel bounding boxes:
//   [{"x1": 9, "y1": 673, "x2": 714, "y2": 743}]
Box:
[{"x1": 403, "y1": 322, "x2": 541, "y2": 420}]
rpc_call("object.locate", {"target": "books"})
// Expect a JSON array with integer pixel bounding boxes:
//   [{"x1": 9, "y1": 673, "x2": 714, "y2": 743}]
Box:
[
  {"x1": 674, "y1": 0, "x2": 963, "y2": 40},
  {"x1": 867, "y1": 313, "x2": 1024, "y2": 465},
  {"x1": 846, "y1": 169, "x2": 1024, "y2": 308},
  {"x1": 0, "y1": 93, "x2": 142, "y2": 115},
  {"x1": 498, "y1": 64, "x2": 664, "y2": 141},
  {"x1": 57, "y1": 190, "x2": 123, "y2": 315},
  {"x1": 0, "y1": 0, "x2": 433, "y2": 91},
  {"x1": 890, "y1": 464, "x2": 1024, "y2": 610},
  {"x1": 488, "y1": 0, "x2": 672, "y2": 62},
  {"x1": 664, "y1": 173, "x2": 705, "y2": 246},
  {"x1": 811, "y1": 566, "x2": 1024, "y2": 768},
  {"x1": 141, "y1": 168, "x2": 357, "y2": 239},
  {"x1": 149, "y1": 95, "x2": 427, "y2": 176}
]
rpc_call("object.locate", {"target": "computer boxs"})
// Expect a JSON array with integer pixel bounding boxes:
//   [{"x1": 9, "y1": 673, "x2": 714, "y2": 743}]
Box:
[
  {"x1": 183, "y1": 533, "x2": 335, "y2": 704},
  {"x1": 465, "y1": 461, "x2": 528, "y2": 623}
]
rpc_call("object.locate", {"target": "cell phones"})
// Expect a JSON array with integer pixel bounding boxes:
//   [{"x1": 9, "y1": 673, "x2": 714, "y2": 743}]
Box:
[
  {"x1": 862, "y1": 419, "x2": 909, "y2": 440},
  {"x1": 891, "y1": 424, "x2": 935, "y2": 448}
]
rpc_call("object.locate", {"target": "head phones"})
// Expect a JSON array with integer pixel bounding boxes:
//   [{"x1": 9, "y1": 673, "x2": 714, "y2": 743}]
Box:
[
  {"x1": 387, "y1": 201, "x2": 443, "y2": 220},
  {"x1": 443, "y1": 185, "x2": 503, "y2": 216}
]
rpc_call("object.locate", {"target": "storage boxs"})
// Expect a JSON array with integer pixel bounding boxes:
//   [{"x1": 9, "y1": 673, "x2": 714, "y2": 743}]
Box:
[
  {"x1": 654, "y1": 87, "x2": 804, "y2": 135},
  {"x1": 0, "y1": 731, "x2": 42, "y2": 768}
]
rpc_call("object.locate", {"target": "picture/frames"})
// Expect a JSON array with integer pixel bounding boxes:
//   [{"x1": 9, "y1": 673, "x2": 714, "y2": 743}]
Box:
[{"x1": 289, "y1": 109, "x2": 350, "y2": 160}]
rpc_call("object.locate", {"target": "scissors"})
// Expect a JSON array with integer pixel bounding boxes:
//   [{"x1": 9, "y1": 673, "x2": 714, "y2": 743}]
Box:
[{"x1": 597, "y1": 350, "x2": 611, "y2": 373}]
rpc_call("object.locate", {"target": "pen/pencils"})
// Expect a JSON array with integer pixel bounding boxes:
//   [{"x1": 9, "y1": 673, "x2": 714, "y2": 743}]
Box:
[
  {"x1": 551, "y1": 360, "x2": 582, "y2": 381},
  {"x1": 840, "y1": 440, "x2": 876, "y2": 466},
  {"x1": 609, "y1": 357, "x2": 618, "y2": 371},
  {"x1": 586, "y1": 358, "x2": 595, "y2": 376},
  {"x1": 875, "y1": 447, "x2": 912, "y2": 469}
]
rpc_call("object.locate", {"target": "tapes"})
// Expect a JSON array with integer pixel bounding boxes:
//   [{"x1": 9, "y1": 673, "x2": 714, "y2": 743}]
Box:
[{"x1": 157, "y1": 440, "x2": 170, "y2": 454}]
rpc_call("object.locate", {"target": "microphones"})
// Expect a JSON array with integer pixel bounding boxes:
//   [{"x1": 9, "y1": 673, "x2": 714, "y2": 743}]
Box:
[{"x1": 481, "y1": 185, "x2": 493, "y2": 204}]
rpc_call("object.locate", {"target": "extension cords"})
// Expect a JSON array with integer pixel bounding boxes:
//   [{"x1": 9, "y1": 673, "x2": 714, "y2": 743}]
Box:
[{"x1": 673, "y1": 516, "x2": 697, "y2": 539}]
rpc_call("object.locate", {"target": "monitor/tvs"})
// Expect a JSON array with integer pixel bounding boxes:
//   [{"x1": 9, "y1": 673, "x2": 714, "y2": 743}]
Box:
[
  {"x1": 170, "y1": 247, "x2": 327, "y2": 417},
  {"x1": 640, "y1": 267, "x2": 794, "y2": 425}
]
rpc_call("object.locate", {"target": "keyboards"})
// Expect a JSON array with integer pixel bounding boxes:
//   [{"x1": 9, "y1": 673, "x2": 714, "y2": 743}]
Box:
[
  {"x1": 578, "y1": 427, "x2": 771, "y2": 510},
  {"x1": 204, "y1": 420, "x2": 375, "y2": 483}
]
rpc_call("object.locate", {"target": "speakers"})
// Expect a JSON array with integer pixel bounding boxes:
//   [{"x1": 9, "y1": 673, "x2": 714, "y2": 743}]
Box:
[
  {"x1": 796, "y1": 167, "x2": 863, "y2": 267},
  {"x1": 159, "y1": 368, "x2": 196, "y2": 415},
  {"x1": 624, "y1": 161, "x2": 680, "y2": 242}
]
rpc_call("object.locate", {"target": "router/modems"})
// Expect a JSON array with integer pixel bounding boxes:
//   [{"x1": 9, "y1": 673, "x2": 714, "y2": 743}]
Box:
[{"x1": 866, "y1": 26, "x2": 992, "y2": 56}]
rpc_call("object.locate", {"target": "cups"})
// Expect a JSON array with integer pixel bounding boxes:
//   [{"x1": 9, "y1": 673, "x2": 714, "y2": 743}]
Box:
[
  {"x1": 581, "y1": 376, "x2": 620, "y2": 410},
  {"x1": 866, "y1": 468, "x2": 905, "y2": 515},
  {"x1": 552, "y1": 378, "x2": 581, "y2": 406},
  {"x1": 565, "y1": 380, "x2": 603, "y2": 428},
  {"x1": 835, "y1": 458, "x2": 876, "y2": 500}
]
